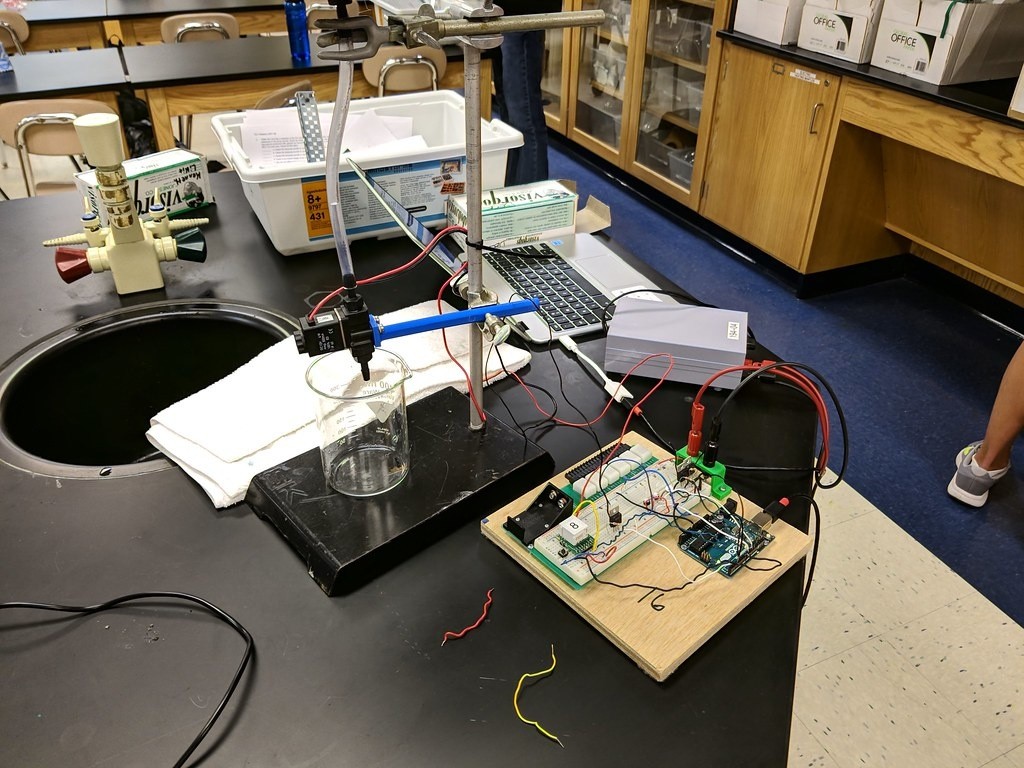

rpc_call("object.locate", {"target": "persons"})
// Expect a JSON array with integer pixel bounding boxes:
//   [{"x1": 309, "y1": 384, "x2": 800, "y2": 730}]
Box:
[
  {"x1": 947, "y1": 339, "x2": 1024, "y2": 508},
  {"x1": 483, "y1": 0, "x2": 563, "y2": 187}
]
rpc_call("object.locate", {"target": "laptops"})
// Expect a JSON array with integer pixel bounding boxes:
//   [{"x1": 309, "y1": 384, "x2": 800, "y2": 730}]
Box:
[{"x1": 346, "y1": 158, "x2": 680, "y2": 344}]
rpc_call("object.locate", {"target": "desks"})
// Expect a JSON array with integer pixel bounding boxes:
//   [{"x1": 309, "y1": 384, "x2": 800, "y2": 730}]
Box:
[
  {"x1": 0, "y1": 45, "x2": 129, "y2": 179},
  {"x1": 122, "y1": 34, "x2": 493, "y2": 155},
  {"x1": 0, "y1": 0, "x2": 106, "y2": 55},
  {"x1": 0, "y1": 170, "x2": 820, "y2": 768},
  {"x1": 106, "y1": 0, "x2": 287, "y2": 42},
  {"x1": 796, "y1": 46, "x2": 1023, "y2": 330}
]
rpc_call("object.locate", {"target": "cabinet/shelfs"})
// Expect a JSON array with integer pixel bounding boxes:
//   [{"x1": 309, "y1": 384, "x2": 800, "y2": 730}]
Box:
[
  {"x1": 491, "y1": 0, "x2": 733, "y2": 214},
  {"x1": 698, "y1": 27, "x2": 908, "y2": 275}
]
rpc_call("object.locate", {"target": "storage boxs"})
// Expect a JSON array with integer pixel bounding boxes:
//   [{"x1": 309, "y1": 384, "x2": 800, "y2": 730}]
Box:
[
  {"x1": 72, "y1": 145, "x2": 215, "y2": 229},
  {"x1": 733, "y1": 0, "x2": 806, "y2": 46},
  {"x1": 797, "y1": 0, "x2": 884, "y2": 65},
  {"x1": 870, "y1": 0, "x2": 1024, "y2": 86},
  {"x1": 444, "y1": 178, "x2": 612, "y2": 256},
  {"x1": 210, "y1": 90, "x2": 526, "y2": 256}
]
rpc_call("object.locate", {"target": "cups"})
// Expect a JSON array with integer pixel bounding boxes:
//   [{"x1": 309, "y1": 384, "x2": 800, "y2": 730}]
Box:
[{"x1": 304, "y1": 347, "x2": 412, "y2": 498}]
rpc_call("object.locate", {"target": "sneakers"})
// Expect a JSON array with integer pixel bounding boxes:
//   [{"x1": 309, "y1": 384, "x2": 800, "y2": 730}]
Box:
[{"x1": 947, "y1": 438, "x2": 1011, "y2": 508}]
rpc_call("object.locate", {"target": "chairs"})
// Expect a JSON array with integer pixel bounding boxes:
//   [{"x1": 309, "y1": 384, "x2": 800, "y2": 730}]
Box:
[
  {"x1": 158, "y1": 13, "x2": 243, "y2": 153},
  {"x1": 0, "y1": 9, "x2": 29, "y2": 56},
  {"x1": 363, "y1": 30, "x2": 447, "y2": 97},
  {"x1": 305, "y1": 0, "x2": 360, "y2": 33},
  {"x1": 0, "y1": 99, "x2": 128, "y2": 198}
]
